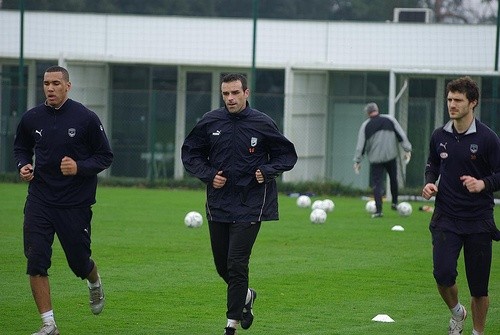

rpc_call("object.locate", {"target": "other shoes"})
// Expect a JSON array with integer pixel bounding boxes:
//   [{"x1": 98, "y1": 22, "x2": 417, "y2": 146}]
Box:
[
  {"x1": 371, "y1": 213, "x2": 381, "y2": 216},
  {"x1": 392, "y1": 204, "x2": 396, "y2": 210}
]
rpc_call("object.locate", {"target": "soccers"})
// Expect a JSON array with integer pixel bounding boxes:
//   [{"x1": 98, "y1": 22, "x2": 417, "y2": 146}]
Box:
[
  {"x1": 184, "y1": 211, "x2": 202, "y2": 229},
  {"x1": 312, "y1": 200, "x2": 324, "y2": 210},
  {"x1": 310, "y1": 209, "x2": 328, "y2": 225},
  {"x1": 398, "y1": 202, "x2": 413, "y2": 216},
  {"x1": 296, "y1": 195, "x2": 311, "y2": 208},
  {"x1": 366, "y1": 201, "x2": 376, "y2": 213},
  {"x1": 324, "y1": 199, "x2": 335, "y2": 212}
]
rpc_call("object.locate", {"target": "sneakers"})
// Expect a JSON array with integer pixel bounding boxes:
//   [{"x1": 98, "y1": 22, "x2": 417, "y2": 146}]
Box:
[
  {"x1": 240, "y1": 288, "x2": 257, "y2": 330},
  {"x1": 31, "y1": 323, "x2": 59, "y2": 335},
  {"x1": 223, "y1": 327, "x2": 237, "y2": 335},
  {"x1": 447, "y1": 305, "x2": 467, "y2": 335},
  {"x1": 87, "y1": 274, "x2": 106, "y2": 315}
]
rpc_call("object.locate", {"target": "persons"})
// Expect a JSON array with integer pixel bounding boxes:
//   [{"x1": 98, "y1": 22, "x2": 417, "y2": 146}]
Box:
[
  {"x1": 353, "y1": 103, "x2": 412, "y2": 218},
  {"x1": 420, "y1": 75, "x2": 500, "y2": 335},
  {"x1": 181, "y1": 73, "x2": 298, "y2": 335},
  {"x1": 14, "y1": 66, "x2": 113, "y2": 335}
]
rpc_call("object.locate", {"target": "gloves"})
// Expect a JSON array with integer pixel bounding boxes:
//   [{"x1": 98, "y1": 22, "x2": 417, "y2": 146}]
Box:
[
  {"x1": 403, "y1": 151, "x2": 412, "y2": 164},
  {"x1": 354, "y1": 162, "x2": 360, "y2": 174}
]
